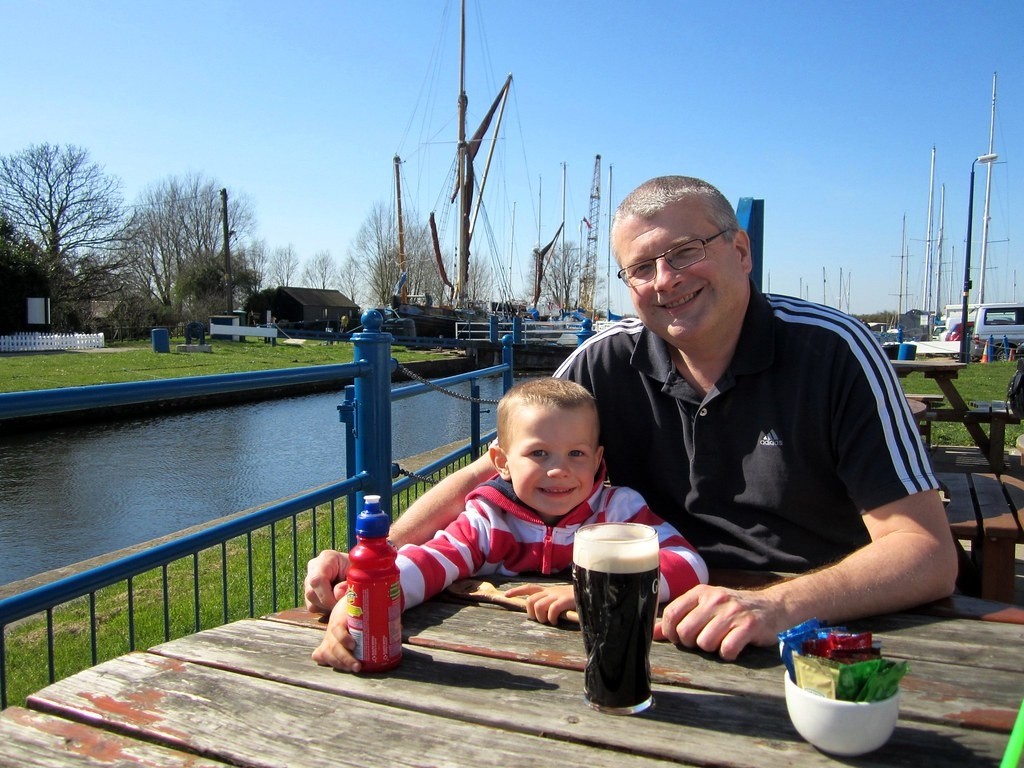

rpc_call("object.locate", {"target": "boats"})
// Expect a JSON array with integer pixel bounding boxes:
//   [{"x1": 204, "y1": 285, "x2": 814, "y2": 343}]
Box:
[{"x1": 374, "y1": 1, "x2": 530, "y2": 350}]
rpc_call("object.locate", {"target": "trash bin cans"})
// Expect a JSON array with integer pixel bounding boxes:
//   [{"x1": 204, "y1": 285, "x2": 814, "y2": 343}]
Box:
[
  {"x1": 152, "y1": 328, "x2": 170, "y2": 352},
  {"x1": 898, "y1": 343, "x2": 917, "y2": 360}
]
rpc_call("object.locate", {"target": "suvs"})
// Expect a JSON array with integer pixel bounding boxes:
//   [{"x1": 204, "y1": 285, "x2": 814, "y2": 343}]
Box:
[{"x1": 945, "y1": 320, "x2": 975, "y2": 359}]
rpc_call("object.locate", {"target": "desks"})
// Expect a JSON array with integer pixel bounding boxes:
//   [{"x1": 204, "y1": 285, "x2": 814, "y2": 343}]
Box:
[
  {"x1": 889, "y1": 359, "x2": 1017, "y2": 478},
  {"x1": 0, "y1": 568, "x2": 1024, "y2": 768}
]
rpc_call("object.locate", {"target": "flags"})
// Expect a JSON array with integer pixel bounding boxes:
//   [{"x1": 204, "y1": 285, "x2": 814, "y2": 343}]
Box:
[{"x1": 397, "y1": 274, "x2": 406, "y2": 294}]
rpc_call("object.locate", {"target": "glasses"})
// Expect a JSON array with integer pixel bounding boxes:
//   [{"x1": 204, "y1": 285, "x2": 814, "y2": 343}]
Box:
[{"x1": 617, "y1": 229, "x2": 726, "y2": 288}]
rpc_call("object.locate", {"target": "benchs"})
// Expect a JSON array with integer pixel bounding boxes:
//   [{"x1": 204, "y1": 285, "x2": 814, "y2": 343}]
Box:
[{"x1": 903, "y1": 395, "x2": 1024, "y2": 603}]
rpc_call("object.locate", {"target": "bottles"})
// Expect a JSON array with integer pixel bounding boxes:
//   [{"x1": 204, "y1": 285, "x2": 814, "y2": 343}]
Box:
[{"x1": 347, "y1": 495, "x2": 402, "y2": 670}]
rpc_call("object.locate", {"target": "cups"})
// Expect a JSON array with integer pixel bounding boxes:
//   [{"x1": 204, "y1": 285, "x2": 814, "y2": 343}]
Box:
[{"x1": 573, "y1": 522, "x2": 660, "y2": 715}]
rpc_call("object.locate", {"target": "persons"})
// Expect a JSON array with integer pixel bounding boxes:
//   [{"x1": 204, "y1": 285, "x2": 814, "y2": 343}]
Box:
[
  {"x1": 311, "y1": 375, "x2": 710, "y2": 675},
  {"x1": 301, "y1": 174, "x2": 959, "y2": 663}
]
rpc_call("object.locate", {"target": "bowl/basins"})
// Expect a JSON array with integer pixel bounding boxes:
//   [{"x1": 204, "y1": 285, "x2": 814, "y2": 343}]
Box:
[{"x1": 783, "y1": 670, "x2": 901, "y2": 756}]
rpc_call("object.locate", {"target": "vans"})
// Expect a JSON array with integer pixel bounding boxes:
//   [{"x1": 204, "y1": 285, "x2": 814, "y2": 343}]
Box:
[{"x1": 970, "y1": 305, "x2": 1024, "y2": 361}]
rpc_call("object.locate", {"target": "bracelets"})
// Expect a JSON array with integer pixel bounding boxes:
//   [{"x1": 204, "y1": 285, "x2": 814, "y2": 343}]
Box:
[{"x1": 388, "y1": 539, "x2": 395, "y2": 548}]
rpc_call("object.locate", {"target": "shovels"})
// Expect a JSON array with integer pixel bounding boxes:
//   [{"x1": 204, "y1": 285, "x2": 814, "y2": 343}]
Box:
[{"x1": 449, "y1": 577, "x2": 580, "y2": 623}]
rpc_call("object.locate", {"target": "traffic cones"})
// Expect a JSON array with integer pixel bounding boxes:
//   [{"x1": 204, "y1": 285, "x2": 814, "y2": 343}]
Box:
[{"x1": 981, "y1": 340, "x2": 988, "y2": 364}]
